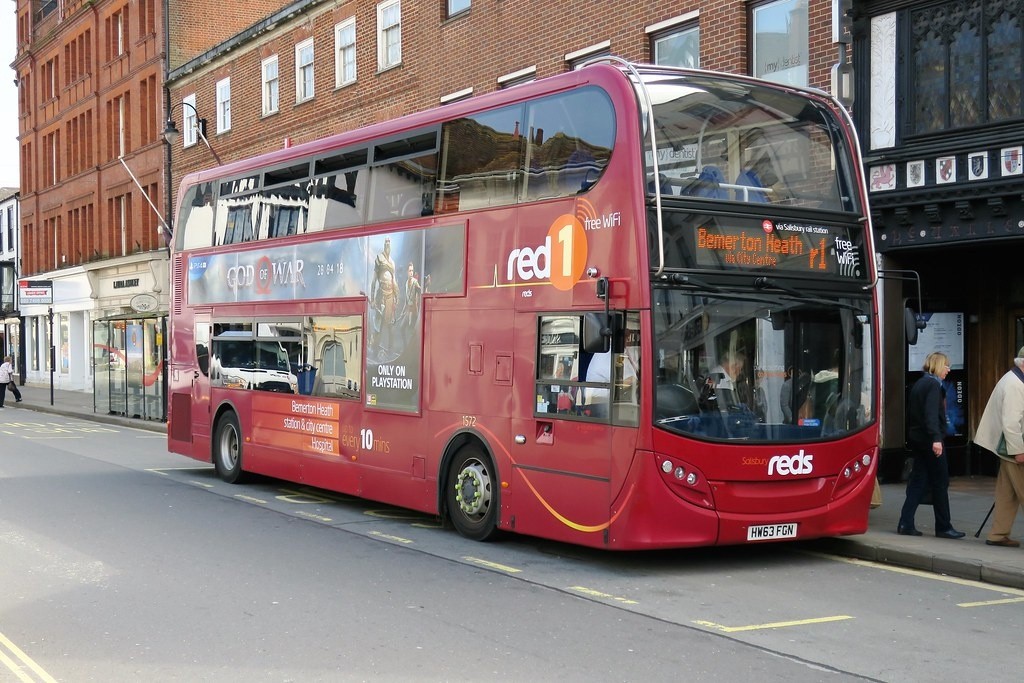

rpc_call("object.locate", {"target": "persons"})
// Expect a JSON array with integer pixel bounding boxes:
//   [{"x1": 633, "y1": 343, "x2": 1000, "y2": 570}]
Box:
[
  {"x1": 974, "y1": 347, "x2": 1024, "y2": 546},
  {"x1": 703, "y1": 351, "x2": 769, "y2": 424},
  {"x1": 898, "y1": 352, "x2": 966, "y2": 538},
  {"x1": 780, "y1": 366, "x2": 793, "y2": 424},
  {"x1": 0, "y1": 356, "x2": 22, "y2": 407},
  {"x1": 543, "y1": 364, "x2": 579, "y2": 415},
  {"x1": 584, "y1": 317, "x2": 639, "y2": 398},
  {"x1": 370, "y1": 237, "x2": 429, "y2": 361}
]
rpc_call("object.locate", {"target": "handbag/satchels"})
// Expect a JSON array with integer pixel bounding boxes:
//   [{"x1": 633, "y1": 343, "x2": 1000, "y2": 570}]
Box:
[
  {"x1": 8, "y1": 383, "x2": 16, "y2": 391},
  {"x1": 996, "y1": 432, "x2": 1015, "y2": 458}
]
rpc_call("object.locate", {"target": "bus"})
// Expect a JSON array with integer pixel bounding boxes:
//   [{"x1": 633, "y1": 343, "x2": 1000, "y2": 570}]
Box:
[{"x1": 167, "y1": 55, "x2": 930, "y2": 552}]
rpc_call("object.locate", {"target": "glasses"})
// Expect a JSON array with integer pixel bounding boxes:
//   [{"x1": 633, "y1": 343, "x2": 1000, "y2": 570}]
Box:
[{"x1": 945, "y1": 365, "x2": 951, "y2": 369}]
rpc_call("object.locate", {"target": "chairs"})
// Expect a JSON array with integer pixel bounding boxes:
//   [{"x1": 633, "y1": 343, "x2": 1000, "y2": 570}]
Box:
[{"x1": 524, "y1": 150, "x2": 767, "y2": 206}]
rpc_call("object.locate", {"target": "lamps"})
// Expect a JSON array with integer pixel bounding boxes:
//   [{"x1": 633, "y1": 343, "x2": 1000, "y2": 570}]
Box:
[
  {"x1": 969, "y1": 310, "x2": 979, "y2": 326},
  {"x1": 158, "y1": 103, "x2": 208, "y2": 148}
]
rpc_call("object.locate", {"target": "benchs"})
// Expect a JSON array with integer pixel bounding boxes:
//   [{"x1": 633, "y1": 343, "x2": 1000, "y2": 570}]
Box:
[{"x1": 110, "y1": 392, "x2": 160, "y2": 405}]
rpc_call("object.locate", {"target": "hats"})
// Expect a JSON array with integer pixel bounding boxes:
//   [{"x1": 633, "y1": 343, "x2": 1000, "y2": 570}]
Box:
[{"x1": 1018, "y1": 346, "x2": 1024, "y2": 358}]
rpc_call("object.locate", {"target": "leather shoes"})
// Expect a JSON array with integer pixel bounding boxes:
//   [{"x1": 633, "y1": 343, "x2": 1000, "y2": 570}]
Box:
[
  {"x1": 898, "y1": 523, "x2": 923, "y2": 536},
  {"x1": 935, "y1": 528, "x2": 965, "y2": 538},
  {"x1": 986, "y1": 537, "x2": 1019, "y2": 546}
]
